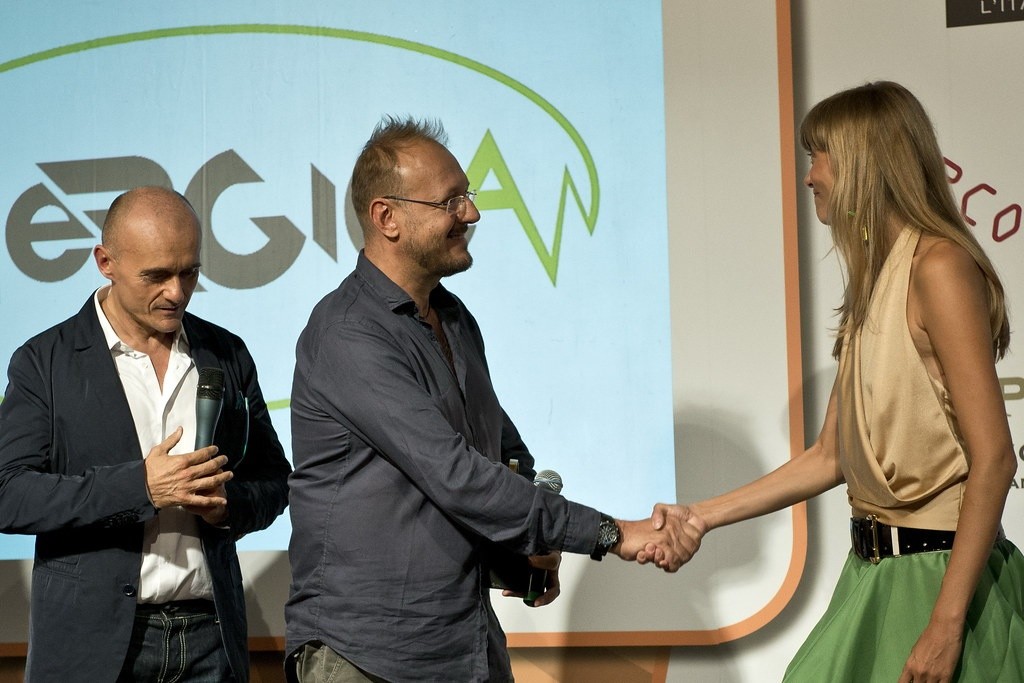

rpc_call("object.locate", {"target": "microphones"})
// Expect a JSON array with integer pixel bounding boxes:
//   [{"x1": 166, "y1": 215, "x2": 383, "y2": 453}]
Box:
[
  {"x1": 526, "y1": 470, "x2": 563, "y2": 603},
  {"x1": 182, "y1": 366, "x2": 224, "y2": 512}
]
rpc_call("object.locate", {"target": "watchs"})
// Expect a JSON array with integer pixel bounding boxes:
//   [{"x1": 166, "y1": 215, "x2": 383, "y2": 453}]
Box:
[{"x1": 591, "y1": 513, "x2": 618, "y2": 561}]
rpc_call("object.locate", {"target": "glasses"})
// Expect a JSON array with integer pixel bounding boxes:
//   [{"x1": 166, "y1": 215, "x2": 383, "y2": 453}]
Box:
[{"x1": 379, "y1": 189, "x2": 476, "y2": 217}]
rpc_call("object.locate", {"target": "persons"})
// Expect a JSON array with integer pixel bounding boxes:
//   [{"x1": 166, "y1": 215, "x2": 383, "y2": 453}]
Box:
[
  {"x1": 285, "y1": 114, "x2": 700, "y2": 683},
  {"x1": 0, "y1": 185, "x2": 292, "y2": 683},
  {"x1": 637, "y1": 81, "x2": 1023, "y2": 683}
]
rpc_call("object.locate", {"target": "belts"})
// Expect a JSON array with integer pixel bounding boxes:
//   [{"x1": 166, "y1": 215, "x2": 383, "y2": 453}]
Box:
[{"x1": 852, "y1": 515, "x2": 955, "y2": 564}]
